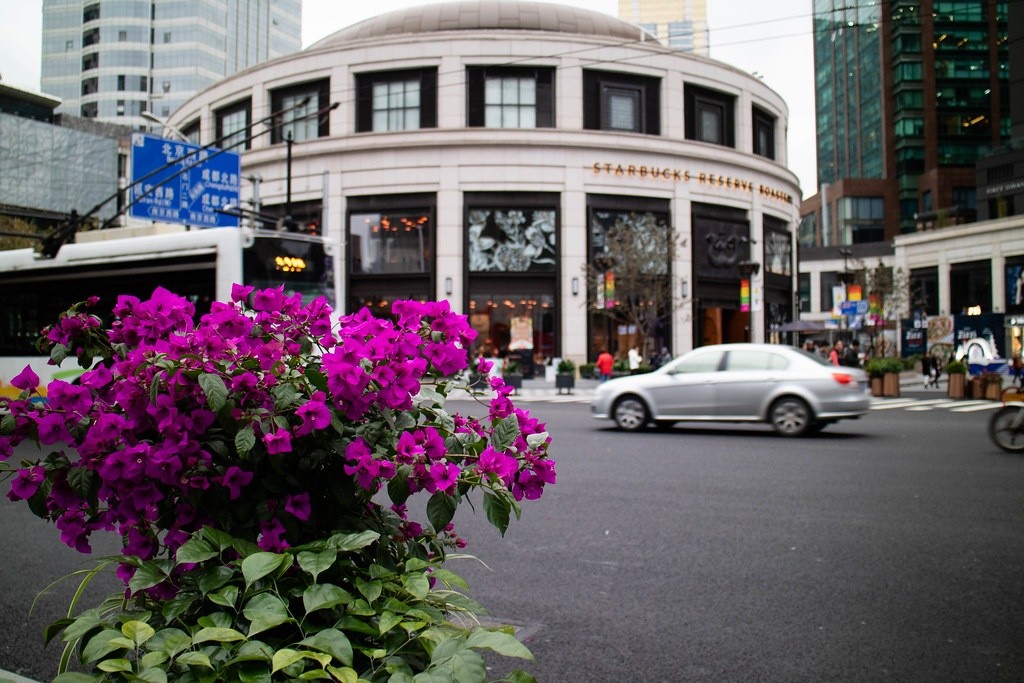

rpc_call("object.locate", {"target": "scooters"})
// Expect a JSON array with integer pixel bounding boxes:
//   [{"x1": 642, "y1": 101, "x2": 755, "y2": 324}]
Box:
[{"x1": 988, "y1": 403, "x2": 1024, "y2": 456}]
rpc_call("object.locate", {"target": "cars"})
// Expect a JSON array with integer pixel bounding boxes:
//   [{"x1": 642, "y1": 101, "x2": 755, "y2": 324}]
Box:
[{"x1": 590, "y1": 343, "x2": 874, "y2": 441}]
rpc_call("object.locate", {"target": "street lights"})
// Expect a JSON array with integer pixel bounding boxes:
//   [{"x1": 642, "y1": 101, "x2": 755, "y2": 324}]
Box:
[{"x1": 138, "y1": 111, "x2": 191, "y2": 146}]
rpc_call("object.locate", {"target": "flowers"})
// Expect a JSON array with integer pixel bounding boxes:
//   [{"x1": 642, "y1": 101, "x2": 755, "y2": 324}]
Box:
[{"x1": 0, "y1": 282, "x2": 556, "y2": 603}]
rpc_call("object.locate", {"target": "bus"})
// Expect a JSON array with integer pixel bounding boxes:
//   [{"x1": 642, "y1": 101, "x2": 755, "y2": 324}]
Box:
[{"x1": 1, "y1": 226, "x2": 337, "y2": 418}]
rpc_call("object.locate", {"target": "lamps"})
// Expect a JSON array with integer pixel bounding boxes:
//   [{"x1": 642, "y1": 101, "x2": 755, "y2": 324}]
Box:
[
  {"x1": 447, "y1": 278, "x2": 452, "y2": 294},
  {"x1": 681, "y1": 281, "x2": 687, "y2": 298},
  {"x1": 572, "y1": 277, "x2": 578, "y2": 295}
]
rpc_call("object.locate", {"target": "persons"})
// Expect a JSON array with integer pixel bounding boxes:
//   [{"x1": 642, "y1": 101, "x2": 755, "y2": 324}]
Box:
[
  {"x1": 922, "y1": 350, "x2": 969, "y2": 389},
  {"x1": 597, "y1": 343, "x2": 670, "y2": 384},
  {"x1": 802, "y1": 339, "x2": 861, "y2": 368}
]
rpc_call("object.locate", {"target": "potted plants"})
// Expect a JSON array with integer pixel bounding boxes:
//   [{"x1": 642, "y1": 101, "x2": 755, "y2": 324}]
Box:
[
  {"x1": 945, "y1": 361, "x2": 965, "y2": 397},
  {"x1": 556, "y1": 359, "x2": 576, "y2": 387},
  {"x1": 505, "y1": 362, "x2": 522, "y2": 387},
  {"x1": 469, "y1": 363, "x2": 488, "y2": 387},
  {"x1": 868, "y1": 358, "x2": 903, "y2": 397}
]
